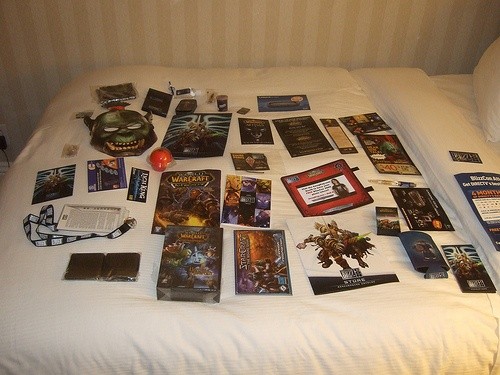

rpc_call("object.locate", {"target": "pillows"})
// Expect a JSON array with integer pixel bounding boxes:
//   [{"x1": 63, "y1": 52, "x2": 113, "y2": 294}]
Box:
[{"x1": 471, "y1": 35, "x2": 500, "y2": 142}]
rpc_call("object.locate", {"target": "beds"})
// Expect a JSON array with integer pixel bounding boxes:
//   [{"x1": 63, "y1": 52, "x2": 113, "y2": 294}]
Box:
[{"x1": 0, "y1": 65, "x2": 500, "y2": 375}]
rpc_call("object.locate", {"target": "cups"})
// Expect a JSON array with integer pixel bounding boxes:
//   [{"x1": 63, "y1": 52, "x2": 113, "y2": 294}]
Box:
[{"x1": 216, "y1": 94, "x2": 228, "y2": 112}]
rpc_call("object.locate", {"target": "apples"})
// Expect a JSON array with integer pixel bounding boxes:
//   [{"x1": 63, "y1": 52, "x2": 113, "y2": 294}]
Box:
[{"x1": 150, "y1": 148, "x2": 171, "y2": 171}]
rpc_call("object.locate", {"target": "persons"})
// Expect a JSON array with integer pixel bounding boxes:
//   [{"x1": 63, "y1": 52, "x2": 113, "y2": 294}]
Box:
[{"x1": 331, "y1": 178, "x2": 351, "y2": 197}]
